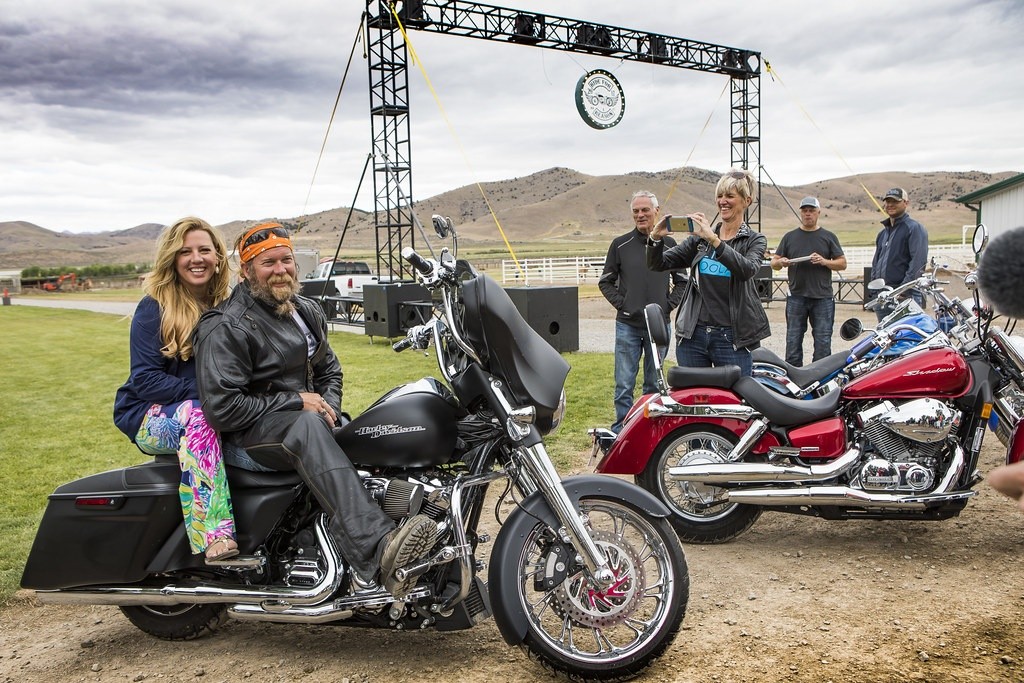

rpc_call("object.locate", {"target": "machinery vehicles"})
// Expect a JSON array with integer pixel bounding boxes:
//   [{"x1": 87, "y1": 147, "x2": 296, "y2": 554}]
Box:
[{"x1": 43, "y1": 271, "x2": 78, "y2": 291}]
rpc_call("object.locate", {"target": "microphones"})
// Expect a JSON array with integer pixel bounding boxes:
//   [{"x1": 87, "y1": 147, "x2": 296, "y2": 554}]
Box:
[{"x1": 977, "y1": 226, "x2": 1024, "y2": 319}]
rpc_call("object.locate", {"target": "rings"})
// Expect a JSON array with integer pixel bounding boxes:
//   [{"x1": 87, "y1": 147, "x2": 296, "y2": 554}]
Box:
[
  {"x1": 700, "y1": 216, "x2": 703, "y2": 222},
  {"x1": 321, "y1": 397, "x2": 327, "y2": 403},
  {"x1": 319, "y1": 408, "x2": 328, "y2": 415}
]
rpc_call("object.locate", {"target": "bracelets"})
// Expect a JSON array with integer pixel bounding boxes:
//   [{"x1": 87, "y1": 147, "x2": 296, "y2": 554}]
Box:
[
  {"x1": 708, "y1": 233, "x2": 718, "y2": 246},
  {"x1": 649, "y1": 231, "x2": 661, "y2": 246}
]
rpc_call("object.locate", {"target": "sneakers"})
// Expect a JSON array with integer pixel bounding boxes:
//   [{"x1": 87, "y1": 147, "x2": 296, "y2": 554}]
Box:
[{"x1": 379, "y1": 515, "x2": 438, "y2": 599}]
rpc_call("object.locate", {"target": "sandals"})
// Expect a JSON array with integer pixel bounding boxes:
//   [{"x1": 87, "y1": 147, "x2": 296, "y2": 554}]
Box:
[{"x1": 205, "y1": 538, "x2": 240, "y2": 561}]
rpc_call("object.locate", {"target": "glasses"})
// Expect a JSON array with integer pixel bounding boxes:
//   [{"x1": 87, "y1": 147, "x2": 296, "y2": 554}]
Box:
[
  {"x1": 242, "y1": 226, "x2": 289, "y2": 252},
  {"x1": 726, "y1": 171, "x2": 754, "y2": 196}
]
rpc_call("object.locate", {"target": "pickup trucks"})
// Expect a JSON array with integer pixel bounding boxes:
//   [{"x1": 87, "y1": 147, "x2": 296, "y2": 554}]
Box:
[{"x1": 304, "y1": 257, "x2": 401, "y2": 315}]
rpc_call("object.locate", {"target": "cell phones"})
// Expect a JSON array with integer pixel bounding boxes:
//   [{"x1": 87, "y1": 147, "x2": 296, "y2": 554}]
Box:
[{"x1": 666, "y1": 216, "x2": 694, "y2": 233}]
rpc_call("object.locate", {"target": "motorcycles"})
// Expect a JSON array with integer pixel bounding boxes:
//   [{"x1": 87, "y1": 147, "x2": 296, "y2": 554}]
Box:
[
  {"x1": 12, "y1": 213, "x2": 691, "y2": 683},
  {"x1": 582, "y1": 222, "x2": 1024, "y2": 546}
]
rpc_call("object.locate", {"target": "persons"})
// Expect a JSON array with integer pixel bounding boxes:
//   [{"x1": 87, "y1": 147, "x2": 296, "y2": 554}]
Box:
[
  {"x1": 195, "y1": 222, "x2": 436, "y2": 602},
  {"x1": 113, "y1": 218, "x2": 240, "y2": 562},
  {"x1": 647, "y1": 168, "x2": 772, "y2": 376},
  {"x1": 771, "y1": 196, "x2": 847, "y2": 367},
  {"x1": 987, "y1": 460, "x2": 1024, "y2": 500},
  {"x1": 598, "y1": 189, "x2": 690, "y2": 443},
  {"x1": 870, "y1": 187, "x2": 929, "y2": 323}
]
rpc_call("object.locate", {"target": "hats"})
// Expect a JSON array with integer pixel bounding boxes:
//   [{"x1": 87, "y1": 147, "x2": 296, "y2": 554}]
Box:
[
  {"x1": 883, "y1": 187, "x2": 908, "y2": 202},
  {"x1": 799, "y1": 197, "x2": 820, "y2": 210}
]
